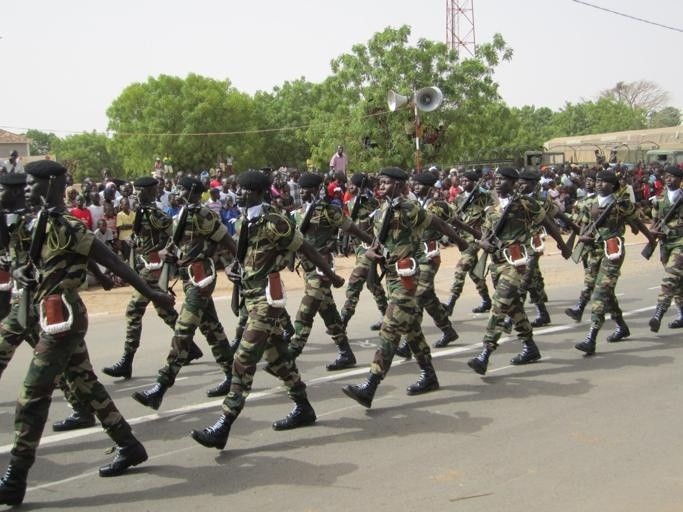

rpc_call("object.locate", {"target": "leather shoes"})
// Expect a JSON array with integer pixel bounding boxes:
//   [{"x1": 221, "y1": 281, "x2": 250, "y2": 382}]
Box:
[{"x1": 52, "y1": 409, "x2": 96, "y2": 432}]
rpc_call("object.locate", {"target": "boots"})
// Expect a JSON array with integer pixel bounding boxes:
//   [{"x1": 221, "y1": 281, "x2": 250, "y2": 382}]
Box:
[
  {"x1": 189, "y1": 412, "x2": 236, "y2": 450},
  {"x1": 101, "y1": 350, "x2": 134, "y2": 380},
  {"x1": 432, "y1": 291, "x2": 683, "y2": 375},
  {"x1": 406, "y1": 364, "x2": 441, "y2": 396},
  {"x1": 340, "y1": 369, "x2": 382, "y2": 410},
  {"x1": 325, "y1": 336, "x2": 357, "y2": 371},
  {"x1": 325, "y1": 314, "x2": 350, "y2": 335},
  {"x1": 228, "y1": 333, "x2": 241, "y2": 351},
  {"x1": 182, "y1": 341, "x2": 204, "y2": 366},
  {"x1": 271, "y1": 396, "x2": 317, "y2": 431},
  {"x1": 99, "y1": 415, "x2": 148, "y2": 477},
  {"x1": 370, "y1": 315, "x2": 384, "y2": 332},
  {"x1": 206, "y1": 374, "x2": 233, "y2": 398},
  {"x1": 130, "y1": 382, "x2": 168, "y2": 412},
  {"x1": 393, "y1": 336, "x2": 413, "y2": 360},
  {"x1": 0, "y1": 463, "x2": 29, "y2": 506}
]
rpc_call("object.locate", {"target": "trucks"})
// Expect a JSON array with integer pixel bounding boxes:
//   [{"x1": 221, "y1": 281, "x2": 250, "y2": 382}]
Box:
[{"x1": 461, "y1": 146, "x2": 571, "y2": 172}]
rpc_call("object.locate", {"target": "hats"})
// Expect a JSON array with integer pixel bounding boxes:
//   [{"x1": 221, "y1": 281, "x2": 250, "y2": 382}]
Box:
[
  {"x1": 133, "y1": 176, "x2": 158, "y2": 187},
  {"x1": 297, "y1": 172, "x2": 323, "y2": 188},
  {"x1": 463, "y1": 171, "x2": 479, "y2": 181},
  {"x1": 518, "y1": 169, "x2": 540, "y2": 181},
  {"x1": 666, "y1": 166, "x2": 683, "y2": 178},
  {"x1": 377, "y1": 166, "x2": 409, "y2": 182},
  {"x1": 22, "y1": 159, "x2": 68, "y2": 180},
  {"x1": 236, "y1": 171, "x2": 274, "y2": 192},
  {"x1": 411, "y1": 174, "x2": 434, "y2": 187},
  {"x1": 595, "y1": 170, "x2": 618, "y2": 184},
  {"x1": 496, "y1": 166, "x2": 519, "y2": 179},
  {"x1": 351, "y1": 172, "x2": 370, "y2": 187},
  {"x1": 585, "y1": 172, "x2": 597, "y2": 181},
  {"x1": 0, "y1": 173, "x2": 27, "y2": 187},
  {"x1": 176, "y1": 176, "x2": 205, "y2": 193}
]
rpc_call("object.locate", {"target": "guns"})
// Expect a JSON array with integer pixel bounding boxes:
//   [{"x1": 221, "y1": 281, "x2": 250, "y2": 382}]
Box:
[
  {"x1": 17, "y1": 175, "x2": 54, "y2": 329},
  {"x1": 129, "y1": 188, "x2": 145, "y2": 272},
  {"x1": 231, "y1": 189, "x2": 253, "y2": 318},
  {"x1": 366, "y1": 181, "x2": 398, "y2": 293},
  {"x1": 286, "y1": 182, "x2": 323, "y2": 272},
  {"x1": 421, "y1": 179, "x2": 683, "y2": 280},
  {"x1": 342, "y1": 177, "x2": 366, "y2": 258},
  {"x1": 157, "y1": 180, "x2": 199, "y2": 293}
]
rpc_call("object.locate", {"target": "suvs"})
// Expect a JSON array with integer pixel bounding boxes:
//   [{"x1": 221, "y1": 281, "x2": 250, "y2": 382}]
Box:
[{"x1": 642, "y1": 150, "x2": 682, "y2": 172}]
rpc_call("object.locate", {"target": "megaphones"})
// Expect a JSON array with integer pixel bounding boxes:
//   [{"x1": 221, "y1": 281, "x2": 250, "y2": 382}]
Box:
[
  {"x1": 386, "y1": 90, "x2": 411, "y2": 112},
  {"x1": 415, "y1": 86, "x2": 443, "y2": 113}
]
